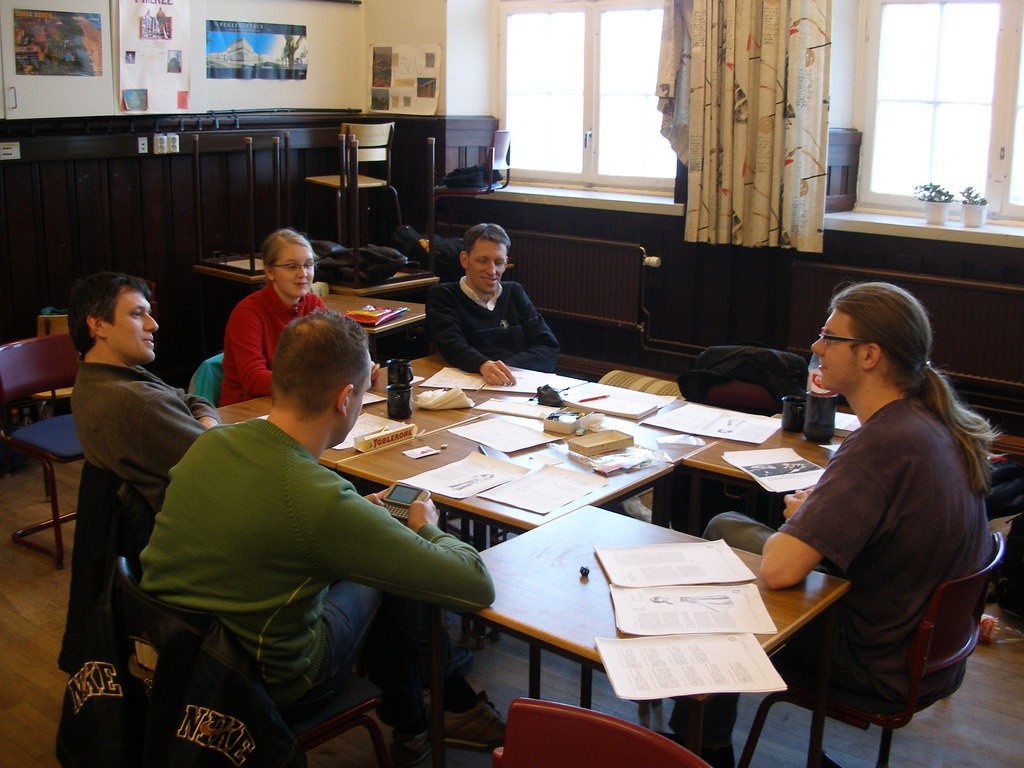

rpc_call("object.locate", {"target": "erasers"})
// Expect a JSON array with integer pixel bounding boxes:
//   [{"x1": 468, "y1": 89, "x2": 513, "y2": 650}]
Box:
[{"x1": 576, "y1": 427, "x2": 585, "y2": 436}]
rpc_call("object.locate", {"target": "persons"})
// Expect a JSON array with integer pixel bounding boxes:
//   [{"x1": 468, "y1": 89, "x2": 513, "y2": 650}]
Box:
[
  {"x1": 67, "y1": 271, "x2": 219, "y2": 510},
  {"x1": 656, "y1": 280, "x2": 988, "y2": 767},
  {"x1": 424, "y1": 224, "x2": 560, "y2": 389},
  {"x1": 216, "y1": 229, "x2": 329, "y2": 409},
  {"x1": 136, "y1": 315, "x2": 511, "y2": 748}
]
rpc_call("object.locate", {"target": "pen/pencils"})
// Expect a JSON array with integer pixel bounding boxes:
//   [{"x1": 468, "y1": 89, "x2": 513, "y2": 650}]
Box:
[
  {"x1": 478, "y1": 445, "x2": 489, "y2": 457},
  {"x1": 577, "y1": 395, "x2": 610, "y2": 403},
  {"x1": 379, "y1": 425, "x2": 388, "y2": 433}
]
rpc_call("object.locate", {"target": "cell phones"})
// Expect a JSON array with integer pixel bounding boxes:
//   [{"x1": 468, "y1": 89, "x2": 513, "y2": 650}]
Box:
[{"x1": 382, "y1": 482, "x2": 430, "y2": 520}]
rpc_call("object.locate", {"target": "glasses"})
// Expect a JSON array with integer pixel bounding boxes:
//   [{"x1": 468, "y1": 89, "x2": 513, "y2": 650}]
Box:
[
  {"x1": 272, "y1": 262, "x2": 313, "y2": 271},
  {"x1": 818, "y1": 327, "x2": 869, "y2": 344}
]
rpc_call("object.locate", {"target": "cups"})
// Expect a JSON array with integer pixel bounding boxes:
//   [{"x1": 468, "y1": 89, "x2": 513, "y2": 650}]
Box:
[
  {"x1": 387, "y1": 358, "x2": 414, "y2": 384},
  {"x1": 386, "y1": 384, "x2": 413, "y2": 419},
  {"x1": 781, "y1": 395, "x2": 805, "y2": 433}
]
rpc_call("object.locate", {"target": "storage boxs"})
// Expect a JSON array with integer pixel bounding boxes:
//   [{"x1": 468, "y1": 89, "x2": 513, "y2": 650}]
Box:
[
  {"x1": 542, "y1": 404, "x2": 594, "y2": 434},
  {"x1": 567, "y1": 429, "x2": 633, "y2": 457}
]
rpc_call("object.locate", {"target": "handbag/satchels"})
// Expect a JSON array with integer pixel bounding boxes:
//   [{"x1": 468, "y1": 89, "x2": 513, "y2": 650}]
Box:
[
  {"x1": 390, "y1": 225, "x2": 431, "y2": 267},
  {"x1": 444, "y1": 164, "x2": 502, "y2": 188},
  {"x1": 311, "y1": 239, "x2": 402, "y2": 289}
]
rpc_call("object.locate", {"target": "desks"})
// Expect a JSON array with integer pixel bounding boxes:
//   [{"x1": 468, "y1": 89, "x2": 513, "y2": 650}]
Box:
[
  {"x1": 213, "y1": 354, "x2": 855, "y2": 768},
  {"x1": 194, "y1": 123, "x2": 441, "y2": 364}
]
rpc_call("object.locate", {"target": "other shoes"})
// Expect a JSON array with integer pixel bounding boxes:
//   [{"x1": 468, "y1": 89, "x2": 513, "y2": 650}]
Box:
[
  {"x1": 444, "y1": 689, "x2": 508, "y2": 751},
  {"x1": 391, "y1": 730, "x2": 430, "y2": 765},
  {"x1": 658, "y1": 732, "x2": 736, "y2": 768}
]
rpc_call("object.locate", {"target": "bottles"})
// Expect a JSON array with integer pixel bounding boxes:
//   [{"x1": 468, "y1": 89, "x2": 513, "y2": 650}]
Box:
[{"x1": 803, "y1": 327, "x2": 836, "y2": 443}]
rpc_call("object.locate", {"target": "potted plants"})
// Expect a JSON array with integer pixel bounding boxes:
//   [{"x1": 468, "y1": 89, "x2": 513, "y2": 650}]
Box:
[
  {"x1": 960, "y1": 186, "x2": 988, "y2": 227},
  {"x1": 911, "y1": 183, "x2": 955, "y2": 225}
]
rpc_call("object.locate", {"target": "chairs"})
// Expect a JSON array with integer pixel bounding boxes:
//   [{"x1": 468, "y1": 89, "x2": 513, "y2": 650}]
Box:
[
  {"x1": 188, "y1": 353, "x2": 221, "y2": 407},
  {"x1": 81, "y1": 463, "x2": 394, "y2": 767},
  {"x1": 424, "y1": 129, "x2": 510, "y2": 234},
  {"x1": 29, "y1": 315, "x2": 75, "y2": 502},
  {"x1": 705, "y1": 379, "x2": 783, "y2": 417},
  {"x1": 305, "y1": 120, "x2": 402, "y2": 245},
  {"x1": 736, "y1": 530, "x2": 1004, "y2": 768},
  {"x1": 493, "y1": 696, "x2": 710, "y2": 768},
  {"x1": 0, "y1": 333, "x2": 84, "y2": 570}
]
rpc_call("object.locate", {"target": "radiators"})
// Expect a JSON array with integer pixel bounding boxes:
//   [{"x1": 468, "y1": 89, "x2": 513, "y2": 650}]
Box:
[
  {"x1": 436, "y1": 223, "x2": 664, "y2": 345},
  {"x1": 787, "y1": 259, "x2": 1024, "y2": 398}
]
